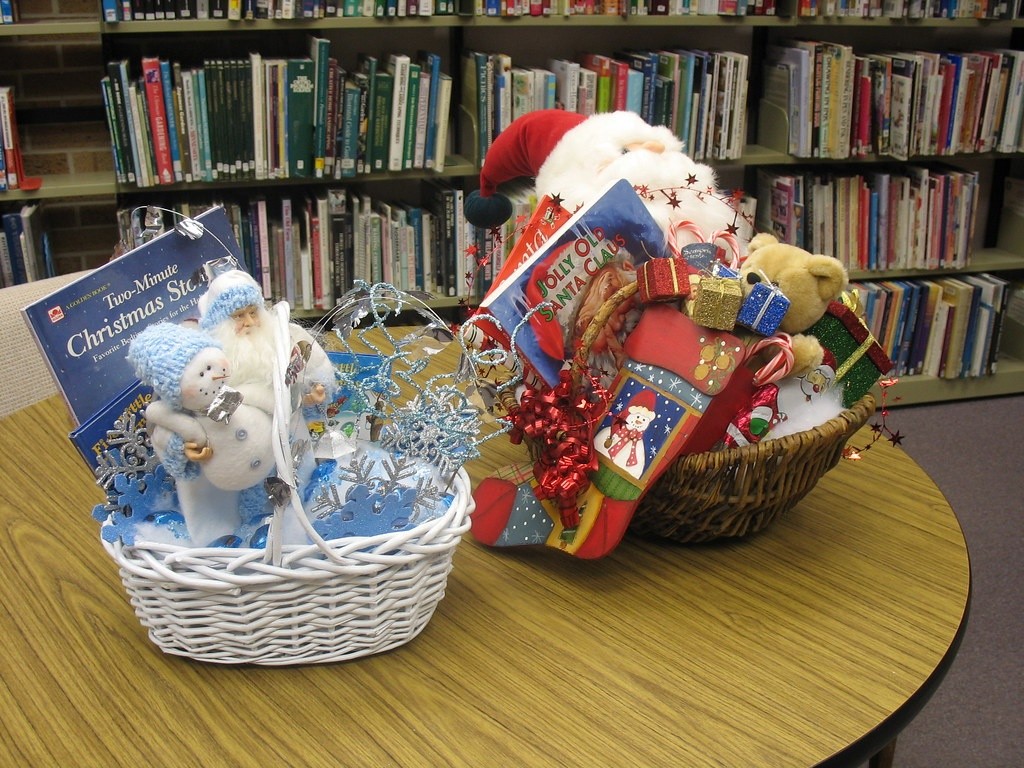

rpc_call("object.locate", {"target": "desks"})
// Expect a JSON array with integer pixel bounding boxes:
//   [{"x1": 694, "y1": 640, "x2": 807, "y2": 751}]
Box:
[{"x1": 0, "y1": 326, "x2": 973, "y2": 768}]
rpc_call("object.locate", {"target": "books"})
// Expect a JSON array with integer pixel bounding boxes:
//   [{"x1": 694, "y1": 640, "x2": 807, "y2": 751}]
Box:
[{"x1": 0, "y1": 0, "x2": 1024, "y2": 495}]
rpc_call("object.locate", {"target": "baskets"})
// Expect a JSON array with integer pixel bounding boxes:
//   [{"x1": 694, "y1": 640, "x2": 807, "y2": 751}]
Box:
[
  {"x1": 493, "y1": 252, "x2": 879, "y2": 545},
  {"x1": 98, "y1": 298, "x2": 478, "y2": 669}
]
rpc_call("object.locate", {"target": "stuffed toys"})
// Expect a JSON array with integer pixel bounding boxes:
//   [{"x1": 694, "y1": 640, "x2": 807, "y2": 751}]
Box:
[{"x1": 127, "y1": 109, "x2": 849, "y2": 560}]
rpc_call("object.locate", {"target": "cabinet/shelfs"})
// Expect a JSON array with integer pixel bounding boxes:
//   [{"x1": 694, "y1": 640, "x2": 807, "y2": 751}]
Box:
[{"x1": 0, "y1": 15, "x2": 1024, "y2": 409}]
